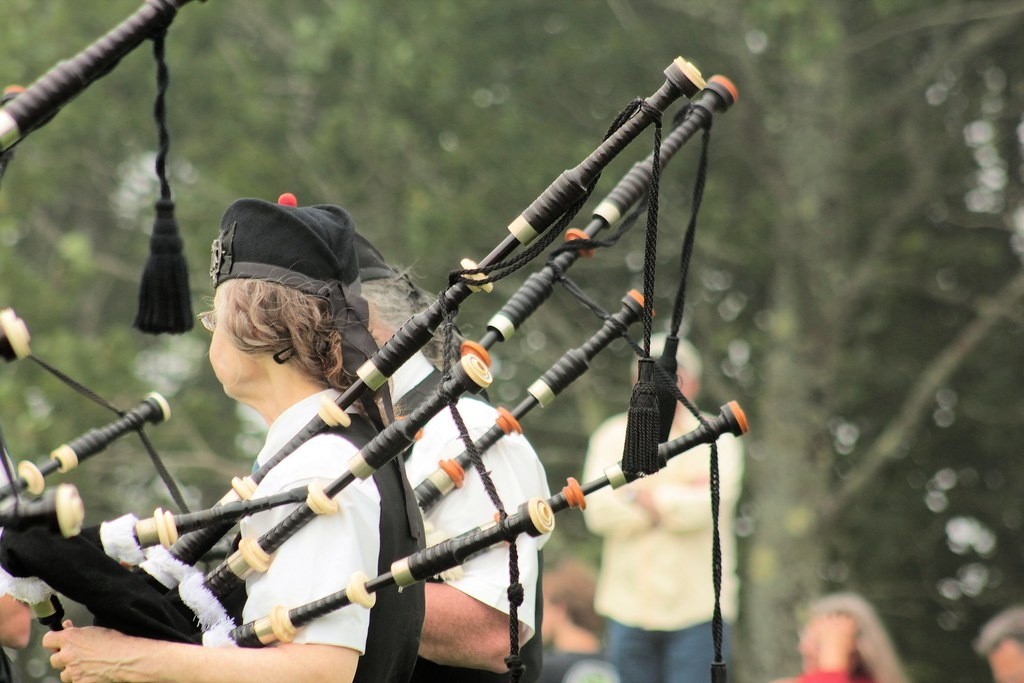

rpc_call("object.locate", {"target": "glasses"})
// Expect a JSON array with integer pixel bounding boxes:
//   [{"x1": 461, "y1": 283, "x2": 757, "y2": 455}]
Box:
[{"x1": 195, "y1": 305, "x2": 234, "y2": 332}]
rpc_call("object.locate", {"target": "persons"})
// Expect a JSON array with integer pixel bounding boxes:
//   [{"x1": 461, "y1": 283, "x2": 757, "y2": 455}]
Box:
[
  {"x1": 353, "y1": 266, "x2": 551, "y2": 683},
  {"x1": 977, "y1": 603, "x2": 1024, "y2": 682},
  {"x1": 799, "y1": 595, "x2": 905, "y2": 683},
  {"x1": 0, "y1": 567, "x2": 32, "y2": 683},
  {"x1": 537, "y1": 564, "x2": 605, "y2": 683},
  {"x1": 583, "y1": 333, "x2": 745, "y2": 683},
  {"x1": 42, "y1": 197, "x2": 426, "y2": 683}
]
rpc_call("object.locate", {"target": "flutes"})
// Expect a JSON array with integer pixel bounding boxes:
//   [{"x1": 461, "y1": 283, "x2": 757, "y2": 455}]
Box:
[{"x1": 0, "y1": 0, "x2": 752, "y2": 647}]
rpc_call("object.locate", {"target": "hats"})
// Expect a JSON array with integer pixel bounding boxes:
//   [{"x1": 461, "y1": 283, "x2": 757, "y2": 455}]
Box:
[
  {"x1": 352, "y1": 233, "x2": 404, "y2": 279},
  {"x1": 210, "y1": 192, "x2": 360, "y2": 295}
]
